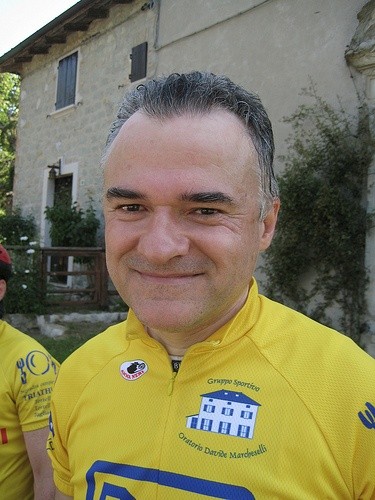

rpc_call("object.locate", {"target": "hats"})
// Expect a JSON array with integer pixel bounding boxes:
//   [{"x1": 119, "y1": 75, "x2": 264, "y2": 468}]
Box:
[{"x1": 0, "y1": 243, "x2": 13, "y2": 265}]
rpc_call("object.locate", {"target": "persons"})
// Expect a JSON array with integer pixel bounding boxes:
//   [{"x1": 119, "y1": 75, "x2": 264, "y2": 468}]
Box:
[
  {"x1": 0, "y1": 246, "x2": 61, "y2": 500},
  {"x1": 47, "y1": 71, "x2": 375, "y2": 500}
]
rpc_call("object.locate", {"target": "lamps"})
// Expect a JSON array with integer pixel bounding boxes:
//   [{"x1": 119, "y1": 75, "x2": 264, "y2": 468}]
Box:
[{"x1": 47, "y1": 159, "x2": 61, "y2": 180}]
